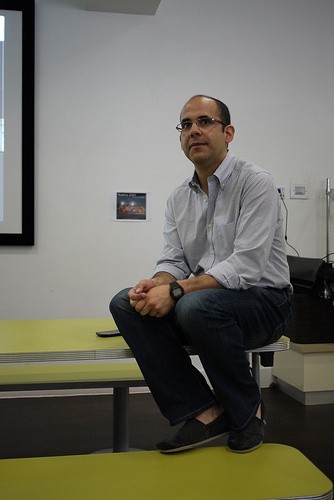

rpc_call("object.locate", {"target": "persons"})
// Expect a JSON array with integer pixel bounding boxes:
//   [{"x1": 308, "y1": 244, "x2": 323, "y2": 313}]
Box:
[{"x1": 108, "y1": 95, "x2": 295, "y2": 454}]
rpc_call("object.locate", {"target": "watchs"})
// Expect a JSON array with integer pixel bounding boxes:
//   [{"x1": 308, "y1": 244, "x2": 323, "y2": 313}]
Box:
[{"x1": 170, "y1": 281, "x2": 185, "y2": 303}]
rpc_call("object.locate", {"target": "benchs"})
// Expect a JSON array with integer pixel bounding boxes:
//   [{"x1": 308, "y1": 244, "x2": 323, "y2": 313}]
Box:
[
  {"x1": 0, "y1": 315, "x2": 290, "y2": 454},
  {"x1": 0, "y1": 443, "x2": 334, "y2": 500}
]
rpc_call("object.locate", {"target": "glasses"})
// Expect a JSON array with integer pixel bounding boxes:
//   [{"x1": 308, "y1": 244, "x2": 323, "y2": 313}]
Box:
[{"x1": 176, "y1": 117, "x2": 227, "y2": 132}]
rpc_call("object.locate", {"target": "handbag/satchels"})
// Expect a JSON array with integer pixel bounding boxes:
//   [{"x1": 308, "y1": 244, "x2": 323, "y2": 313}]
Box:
[{"x1": 286, "y1": 254, "x2": 334, "y2": 343}]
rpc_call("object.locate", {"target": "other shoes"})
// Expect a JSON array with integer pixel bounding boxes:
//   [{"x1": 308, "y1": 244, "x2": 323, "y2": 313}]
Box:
[
  {"x1": 156, "y1": 412, "x2": 229, "y2": 453},
  {"x1": 228, "y1": 400, "x2": 267, "y2": 453}
]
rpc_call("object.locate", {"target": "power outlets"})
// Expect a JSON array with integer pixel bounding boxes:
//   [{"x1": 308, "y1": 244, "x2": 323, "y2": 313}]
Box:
[{"x1": 277, "y1": 185, "x2": 286, "y2": 200}]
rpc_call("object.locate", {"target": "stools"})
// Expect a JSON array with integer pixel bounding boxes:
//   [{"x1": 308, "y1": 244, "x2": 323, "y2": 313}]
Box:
[{"x1": 269, "y1": 341, "x2": 334, "y2": 405}]
